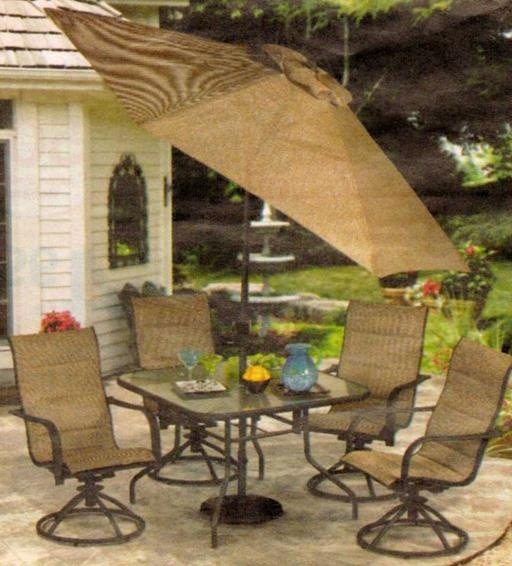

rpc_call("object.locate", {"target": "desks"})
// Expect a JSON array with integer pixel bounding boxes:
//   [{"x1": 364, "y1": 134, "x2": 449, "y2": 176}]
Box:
[{"x1": 116, "y1": 352, "x2": 375, "y2": 550}]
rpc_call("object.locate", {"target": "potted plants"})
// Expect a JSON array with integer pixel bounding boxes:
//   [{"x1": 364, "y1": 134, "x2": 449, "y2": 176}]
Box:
[{"x1": 439, "y1": 280, "x2": 491, "y2": 323}]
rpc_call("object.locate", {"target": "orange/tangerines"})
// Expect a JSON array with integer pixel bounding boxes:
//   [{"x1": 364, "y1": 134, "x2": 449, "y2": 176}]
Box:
[{"x1": 244, "y1": 366, "x2": 270, "y2": 381}]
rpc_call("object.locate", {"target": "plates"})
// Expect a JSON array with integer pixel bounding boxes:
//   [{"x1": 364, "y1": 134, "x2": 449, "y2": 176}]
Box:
[{"x1": 175, "y1": 380, "x2": 227, "y2": 393}]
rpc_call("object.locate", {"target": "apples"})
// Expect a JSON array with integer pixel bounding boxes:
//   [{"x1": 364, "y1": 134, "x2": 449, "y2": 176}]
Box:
[{"x1": 249, "y1": 353, "x2": 285, "y2": 368}]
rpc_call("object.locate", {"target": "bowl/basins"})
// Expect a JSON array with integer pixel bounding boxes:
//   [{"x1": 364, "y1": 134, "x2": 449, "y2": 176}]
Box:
[{"x1": 242, "y1": 375, "x2": 271, "y2": 395}]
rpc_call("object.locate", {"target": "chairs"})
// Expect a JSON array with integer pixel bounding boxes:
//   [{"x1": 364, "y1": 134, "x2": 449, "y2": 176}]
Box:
[
  {"x1": 127, "y1": 292, "x2": 272, "y2": 482},
  {"x1": 287, "y1": 296, "x2": 432, "y2": 506},
  {"x1": 6, "y1": 325, "x2": 164, "y2": 546},
  {"x1": 343, "y1": 335, "x2": 511, "y2": 556}
]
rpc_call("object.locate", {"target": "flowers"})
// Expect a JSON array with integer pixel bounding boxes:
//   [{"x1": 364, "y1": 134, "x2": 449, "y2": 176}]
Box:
[
  {"x1": 377, "y1": 241, "x2": 494, "y2": 300},
  {"x1": 36, "y1": 307, "x2": 83, "y2": 332}
]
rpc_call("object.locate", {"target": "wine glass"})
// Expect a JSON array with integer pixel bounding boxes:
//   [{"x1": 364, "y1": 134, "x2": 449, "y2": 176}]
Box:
[{"x1": 178, "y1": 352, "x2": 223, "y2": 389}]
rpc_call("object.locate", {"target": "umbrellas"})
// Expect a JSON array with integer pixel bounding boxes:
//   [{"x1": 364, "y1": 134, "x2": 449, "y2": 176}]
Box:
[{"x1": 43, "y1": 5, "x2": 471, "y2": 497}]
